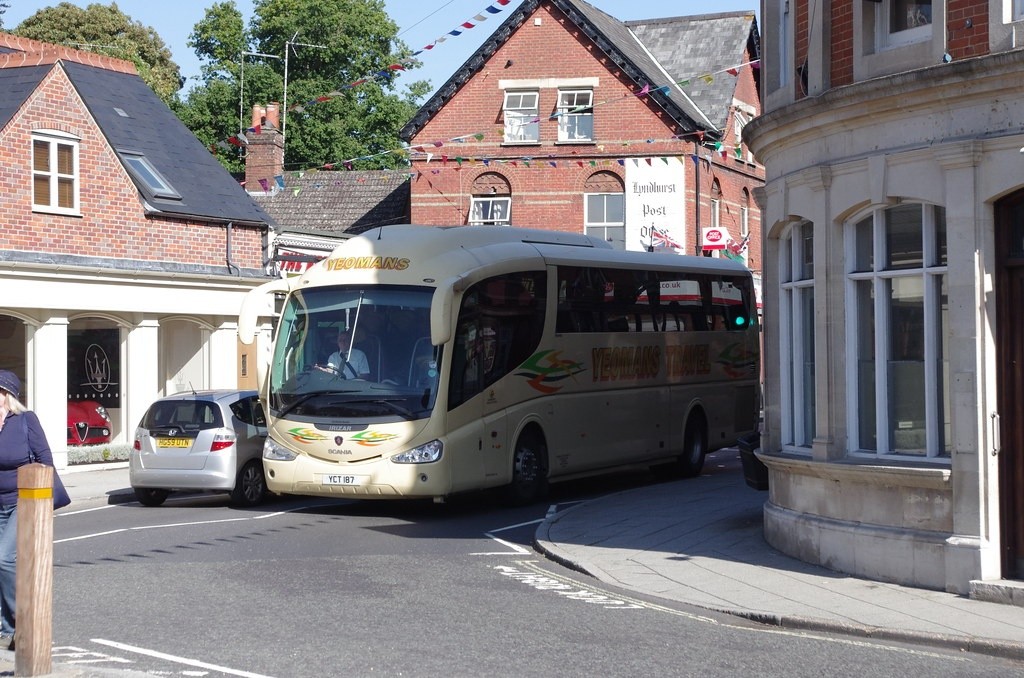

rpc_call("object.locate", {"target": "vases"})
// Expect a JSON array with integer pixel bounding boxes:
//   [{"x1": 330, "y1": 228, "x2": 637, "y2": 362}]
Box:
[{"x1": 736, "y1": 432, "x2": 769, "y2": 491}]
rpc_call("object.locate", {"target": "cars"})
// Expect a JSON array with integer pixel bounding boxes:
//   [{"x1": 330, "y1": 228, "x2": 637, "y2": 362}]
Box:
[
  {"x1": 67, "y1": 400, "x2": 114, "y2": 446},
  {"x1": 129, "y1": 387, "x2": 271, "y2": 508}
]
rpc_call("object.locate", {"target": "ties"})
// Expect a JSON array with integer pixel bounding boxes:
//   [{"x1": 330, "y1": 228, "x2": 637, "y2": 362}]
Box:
[{"x1": 337, "y1": 354, "x2": 347, "y2": 376}]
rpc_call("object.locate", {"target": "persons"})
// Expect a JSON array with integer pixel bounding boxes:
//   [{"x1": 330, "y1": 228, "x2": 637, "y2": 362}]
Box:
[
  {"x1": 0, "y1": 369, "x2": 70, "y2": 651},
  {"x1": 314, "y1": 331, "x2": 371, "y2": 381}
]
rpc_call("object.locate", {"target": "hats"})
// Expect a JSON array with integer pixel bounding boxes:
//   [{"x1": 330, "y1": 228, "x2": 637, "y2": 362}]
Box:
[{"x1": 0, "y1": 369, "x2": 22, "y2": 398}]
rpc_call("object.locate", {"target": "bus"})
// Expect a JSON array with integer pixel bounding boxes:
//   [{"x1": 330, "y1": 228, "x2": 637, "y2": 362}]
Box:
[{"x1": 237, "y1": 221, "x2": 762, "y2": 509}]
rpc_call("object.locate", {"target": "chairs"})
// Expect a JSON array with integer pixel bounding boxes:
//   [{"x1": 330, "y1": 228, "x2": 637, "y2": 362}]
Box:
[
  {"x1": 254, "y1": 403, "x2": 266, "y2": 427},
  {"x1": 408, "y1": 336, "x2": 440, "y2": 387},
  {"x1": 350, "y1": 325, "x2": 385, "y2": 382},
  {"x1": 607, "y1": 306, "x2": 746, "y2": 332}
]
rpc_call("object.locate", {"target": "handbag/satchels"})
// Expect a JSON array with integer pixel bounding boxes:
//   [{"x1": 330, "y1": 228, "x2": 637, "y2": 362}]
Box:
[{"x1": 52, "y1": 465, "x2": 71, "y2": 510}]
